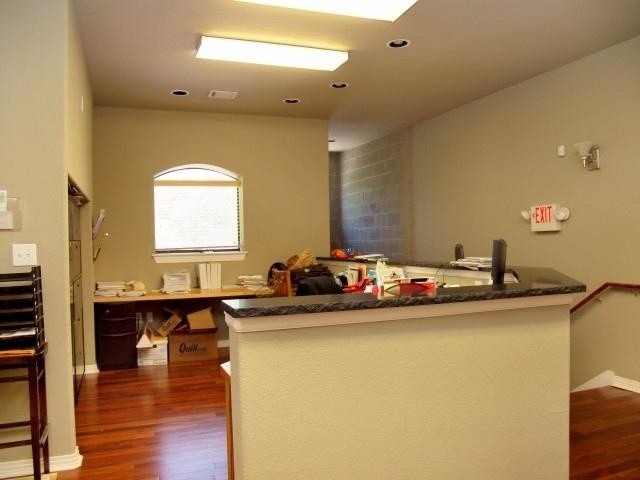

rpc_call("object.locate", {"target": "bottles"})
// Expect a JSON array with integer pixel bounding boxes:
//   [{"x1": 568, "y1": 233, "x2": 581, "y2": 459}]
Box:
[{"x1": 454, "y1": 240, "x2": 464, "y2": 261}]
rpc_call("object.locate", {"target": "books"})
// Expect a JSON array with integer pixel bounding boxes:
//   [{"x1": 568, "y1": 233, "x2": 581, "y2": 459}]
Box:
[{"x1": 195, "y1": 263, "x2": 221, "y2": 289}]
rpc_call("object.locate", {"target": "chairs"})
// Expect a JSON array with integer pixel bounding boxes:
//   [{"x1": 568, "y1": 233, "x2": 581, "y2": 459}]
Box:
[
  {"x1": 296, "y1": 276, "x2": 344, "y2": 296},
  {"x1": 272, "y1": 268, "x2": 292, "y2": 297}
]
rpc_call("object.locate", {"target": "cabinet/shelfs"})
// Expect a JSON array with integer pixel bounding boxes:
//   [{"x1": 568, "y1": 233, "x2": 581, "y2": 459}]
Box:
[{"x1": 94, "y1": 285, "x2": 274, "y2": 370}]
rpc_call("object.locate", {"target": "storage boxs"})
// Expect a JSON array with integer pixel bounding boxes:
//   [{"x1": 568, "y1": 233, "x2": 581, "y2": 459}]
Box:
[
  {"x1": 168, "y1": 327, "x2": 218, "y2": 363},
  {"x1": 153, "y1": 307, "x2": 184, "y2": 337},
  {"x1": 187, "y1": 306, "x2": 216, "y2": 329}
]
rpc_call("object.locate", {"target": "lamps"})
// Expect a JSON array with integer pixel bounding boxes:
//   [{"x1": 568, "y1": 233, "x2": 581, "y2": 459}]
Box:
[
  {"x1": 573, "y1": 141, "x2": 600, "y2": 170},
  {"x1": 195, "y1": 35, "x2": 349, "y2": 72}
]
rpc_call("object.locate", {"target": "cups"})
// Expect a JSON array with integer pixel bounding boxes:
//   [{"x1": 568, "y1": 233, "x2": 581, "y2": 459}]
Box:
[{"x1": 343, "y1": 247, "x2": 354, "y2": 258}]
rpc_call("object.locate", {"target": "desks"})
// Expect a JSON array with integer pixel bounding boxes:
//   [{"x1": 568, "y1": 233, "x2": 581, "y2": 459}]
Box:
[{"x1": 0, "y1": 342, "x2": 49, "y2": 480}]
